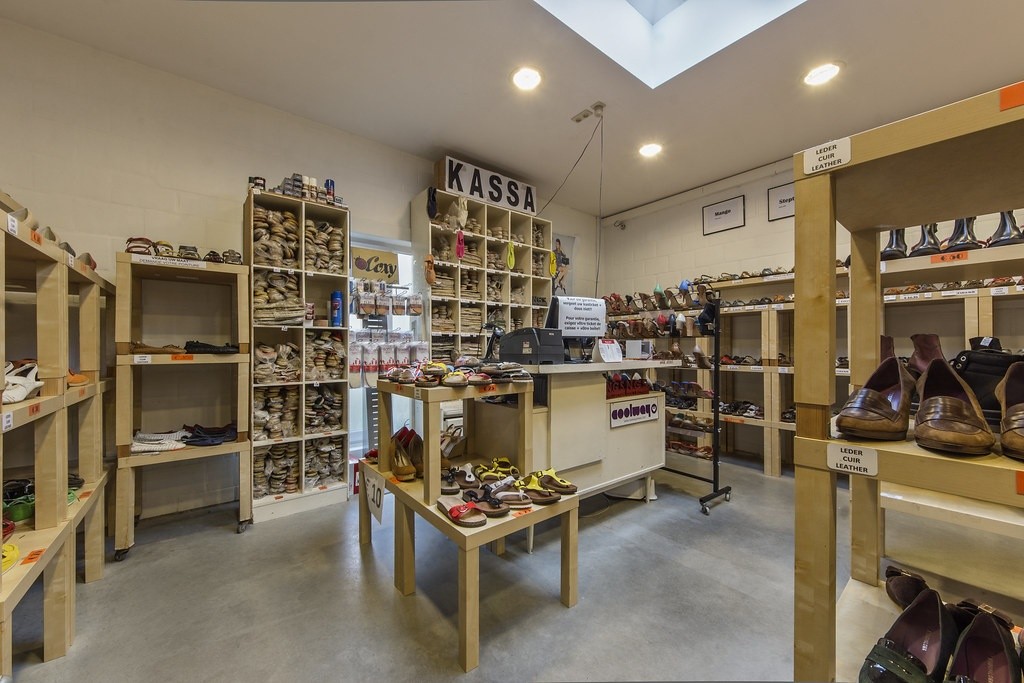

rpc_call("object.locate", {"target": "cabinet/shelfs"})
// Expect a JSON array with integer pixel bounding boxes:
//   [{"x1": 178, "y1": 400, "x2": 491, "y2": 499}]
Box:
[
  {"x1": 0, "y1": 210, "x2": 116, "y2": 683},
  {"x1": 793, "y1": 80, "x2": 1024, "y2": 683},
  {"x1": 606, "y1": 243, "x2": 1023, "y2": 483},
  {"x1": 113, "y1": 252, "x2": 252, "y2": 562},
  {"x1": 242, "y1": 189, "x2": 350, "y2": 525},
  {"x1": 410, "y1": 187, "x2": 553, "y2": 363},
  {"x1": 358, "y1": 378, "x2": 579, "y2": 674}
]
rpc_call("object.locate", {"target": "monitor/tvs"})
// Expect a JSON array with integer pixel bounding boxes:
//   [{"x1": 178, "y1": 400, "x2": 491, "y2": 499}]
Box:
[{"x1": 545, "y1": 297, "x2": 603, "y2": 364}]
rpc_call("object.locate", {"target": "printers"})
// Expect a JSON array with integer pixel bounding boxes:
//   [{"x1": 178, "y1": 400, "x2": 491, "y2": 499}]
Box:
[{"x1": 499, "y1": 327, "x2": 565, "y2": 366}]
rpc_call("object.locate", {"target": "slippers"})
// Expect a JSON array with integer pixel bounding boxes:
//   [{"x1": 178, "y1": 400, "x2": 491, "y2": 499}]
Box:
[
  {"x1": 693, "y1": 257, "x2": 1023, "y2": 307},
  {"x1": 439, "y1": 457, "x2": 576, "y2": 528},
  {"x1": 720, "y1": 351, "x2": 848, "y2": 369},
  {"x1": 387, "y1": 353, "x2": 533, "y2": 387}
]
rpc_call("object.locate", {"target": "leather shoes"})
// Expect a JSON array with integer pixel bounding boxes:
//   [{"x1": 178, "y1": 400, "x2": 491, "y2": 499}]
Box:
[
  {"x1": 858, "y1": 565, "x2": 1024, "y2": 683},
  {"x1": 880, "y1": 208, "x2": 1023, "y2": 260}
]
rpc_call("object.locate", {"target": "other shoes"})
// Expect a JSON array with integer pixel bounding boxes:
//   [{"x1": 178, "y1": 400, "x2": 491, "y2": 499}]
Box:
[
  {"x1": 718, "y1": 401, "x2": 796, "y2": 423},
  {"x1": 3, "y1": 471, "x2": 84, "y2": 575},
  {"x1": 131, "y1": 423, "x2": 238, "y2": 454},
  {"x1": 132, "y1": 340, "x2": 238, "y2": 354},
  {"x1": 423, "y1": 186, "x2": 558, "y2": 363},
  {"x1": 3, "y1": 359, "x2": 91, "y2": 405},
  {"x1": 831, "y1": 334, "x2": 1024, "y2": 460},
  {"x1": 252, "y1": 204, "x2": 345, "y2": 498}
]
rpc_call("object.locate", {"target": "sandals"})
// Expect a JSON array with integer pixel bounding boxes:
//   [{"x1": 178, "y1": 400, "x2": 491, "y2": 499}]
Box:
[{"x1": 125, "y1": 237, "x2": 243, "y2": 266}]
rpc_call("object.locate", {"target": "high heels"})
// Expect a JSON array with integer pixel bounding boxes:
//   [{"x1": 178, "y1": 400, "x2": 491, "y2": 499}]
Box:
[
  {"x1": 364, "y1": 421, "x2": 467, "y2": 481},
  {"x1": 577, "y1": 280, "x2": 713, "y2": 460}
]
